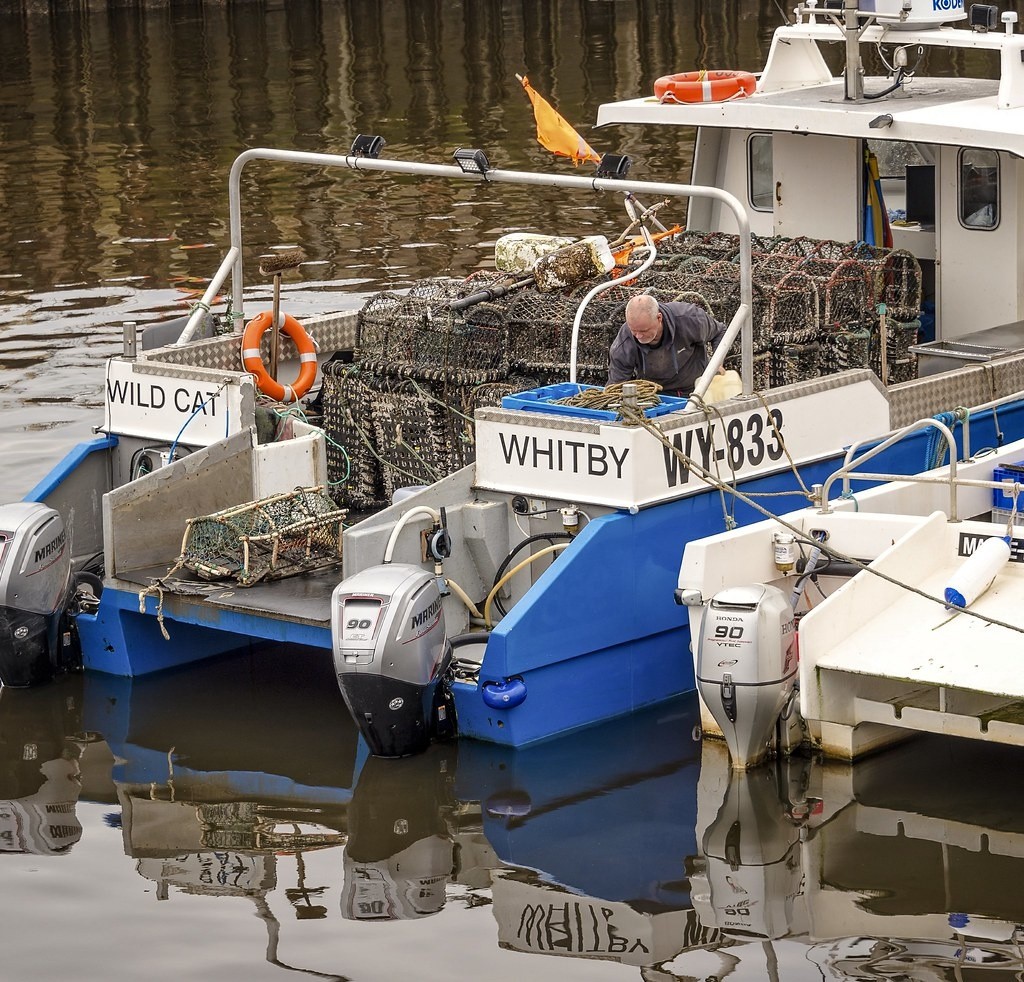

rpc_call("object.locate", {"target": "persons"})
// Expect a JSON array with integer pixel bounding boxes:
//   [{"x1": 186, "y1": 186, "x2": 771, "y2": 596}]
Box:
[{"x1": 605, "y1": 296, "x2": 728, "y2": 398}]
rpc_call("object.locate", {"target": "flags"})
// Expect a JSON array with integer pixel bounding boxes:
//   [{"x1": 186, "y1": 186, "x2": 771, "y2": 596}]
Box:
[
  {"x1": 524, "y1": 76, "x2": 602, "y2": 167},
  {"x1": 609, "y1": 226, "x2": 683, "y2": 286}
]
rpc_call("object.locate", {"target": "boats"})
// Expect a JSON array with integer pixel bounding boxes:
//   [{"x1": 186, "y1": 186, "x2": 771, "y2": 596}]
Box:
[
  {"x1": 0, "y1": 672, "x2": 692, "y2": 980},
  {"x1": 0, "y1": 0, "x2": 1024, "y2": 755},
  {"x1": 666, "y1": 430, "x2": 1024, "y2": 762},
  {"x1": 490, "y1": 759, "x2": 1023, "y2": 982}
]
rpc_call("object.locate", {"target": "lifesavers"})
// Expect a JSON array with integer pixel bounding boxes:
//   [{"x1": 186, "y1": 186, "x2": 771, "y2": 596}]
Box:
[
  {"x1": 653, "y1": 69, "x2": 757, "y2": 103},
  {"x1": 241, "y1": 309, "x2": 319, "y2": 404}
]
